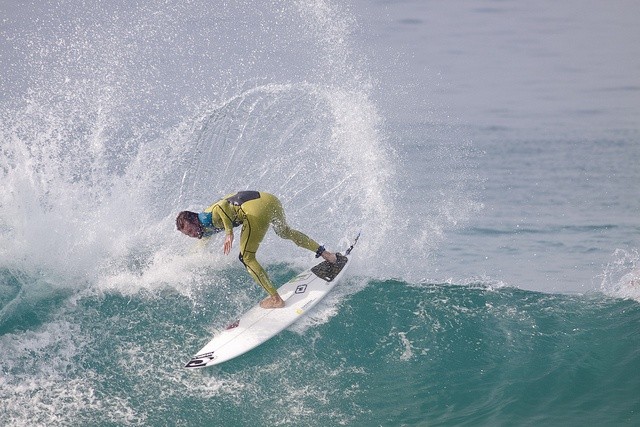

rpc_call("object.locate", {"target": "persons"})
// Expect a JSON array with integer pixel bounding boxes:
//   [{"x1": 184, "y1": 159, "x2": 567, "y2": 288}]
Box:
[{"x1": 174, "y1": 189, "x2": 339, "y2": 308}]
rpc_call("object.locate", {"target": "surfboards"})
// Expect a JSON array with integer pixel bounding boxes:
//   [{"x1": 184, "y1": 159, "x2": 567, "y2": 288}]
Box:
[{"x1": 183, "y1": 249, "x2": 351, "y2": 369}]
